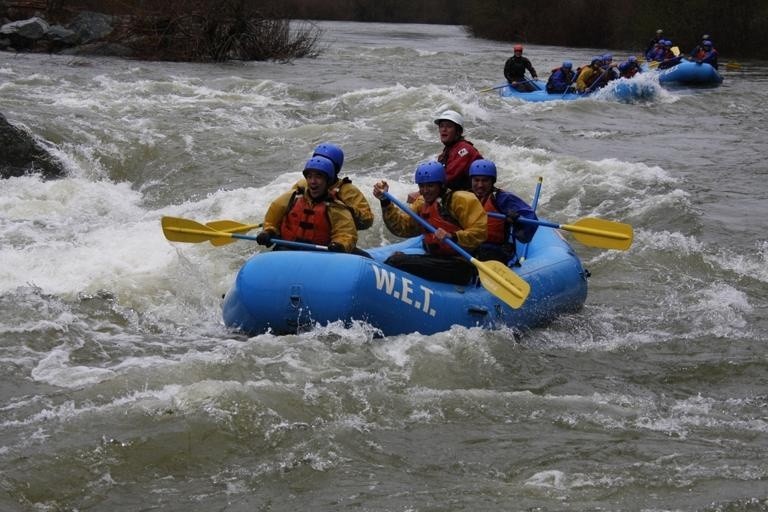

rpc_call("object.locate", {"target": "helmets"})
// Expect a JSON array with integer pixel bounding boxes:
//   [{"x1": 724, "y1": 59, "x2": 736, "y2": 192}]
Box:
[
  {"x1": 514, "y1": 44, "x2": 523, "y2": 53},
  {"x1": 654, "y1": 44, "x2": 658, "y2": 47},
  {"x1": 433, "y1": 109, "x2": 465, "y2": 129},
  {"x1": 562, "y1": 62, "x2": 572, "y2": 69},
  {"x1": 656, "y1": 29, "x2": 663, "y2": 34},
  {"x1": 591, "y1": 56, "x2": 602, "y2": 63},
  {"x1": 627, "y1": 56, "x2": 638, "y2": 62},
  {"x1": 603, "y1": 55, "x2": 613, "y2": 60},
  {"x1": 703, "y1": 40, "x2": 712, "y2": 46},
  {"x1": 702, "y1": 34, "x2": 710, "y2": 40},
  {"x1": 664, "y1": 41, "x2": 672, "y2": 46},
  {"x1": 468, "y1": 159, "x2": 497, "y2": 178},
  {"x1": 414, "y1": 160, "x2": 446, "y2": 184},
  {"x1": 659, "y1": 39, "x2": 665, "y2": 43},
  {"x1": 312, "y1": 143, "x2": 344, "y2": 174},
  {"x1": 301, "y1": 155, "x2": 339, "y2": 187},
  {"x1": 619, "y1": 61, "x2": 629, "y2": 71}
]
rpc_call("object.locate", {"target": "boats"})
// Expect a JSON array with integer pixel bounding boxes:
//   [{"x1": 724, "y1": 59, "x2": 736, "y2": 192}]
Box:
[
  {"x1": 222, "y1": 216, "x2": 588, "y2": 336},
  {"x1": 498, "y1": 79, "x2": 656, "y2": 102},
  {"x1": 640, "y1": 56, "x2": 724, "y2": 86}
]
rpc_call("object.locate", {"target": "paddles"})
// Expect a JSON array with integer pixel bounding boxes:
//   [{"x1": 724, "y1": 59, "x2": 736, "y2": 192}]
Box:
[
  {"x1": 160, "y1": 216, "x2": 329, "y2": 251},
  {"x1": 205, "y1": 219, "x2": 264, "y2": 246},
  {"x1": 377, "y1": 180, "x2": 531, "y2": 310},
  {"x1": 486, "y1": 210, "x2": 634, "y2": 250},
  {"x1": 647, "y1": 55, "x2": 685, "y2": 70},
  {"x1": 636, "y1": 57, "x2": 653, "y2": 64}
]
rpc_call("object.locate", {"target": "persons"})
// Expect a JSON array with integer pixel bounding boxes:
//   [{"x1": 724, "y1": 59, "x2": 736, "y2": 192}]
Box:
[
  {"x1": 603, "y1": 53, "x2": 621, "y2": 80},
  {"x1": 696, "y1": 40, "x2": 718, "y2": 70},
  {"x1": 619, "y1": 56, "x2": 643, "y2": 80},
  {"x1": 406, "y1": 110, "x2": 485, "y2": 203},
  {"x1": 504, "y1": 46, "x2": 538, "y2": 93},
  {"x1": 469, "y1": 160, "x2": 538, "y2": 271},
  {"x1": 290, "y1": 144, "x2": 373, "y2": 230},
  {"x1": 256, "y1": 156, "x2": 358, "y2": 252},
  {"x1": 644, "y1": 29, "x2": 662, "y2": 57},
  {"x1": 546, "y1": 61, "x2": 581, "y2": 94},
  {"x1": 688, "y1": 34, "x2": 711, "y2": 61},
  {"x1": 658, "y1": 39, "x2": 665, "y2": 47},
  {"x1": 647, "y1": 44, "x2": 665, "y2": 61},
  {"x1": 577, "y1": 56, "x2": 618, "y2": 93},
  {"x1": 664, "y1": 41, "x2": 675, "y2": 58},
  {"x1": 374, "y1": 160, "x2": 489, "y2": 285}
]
